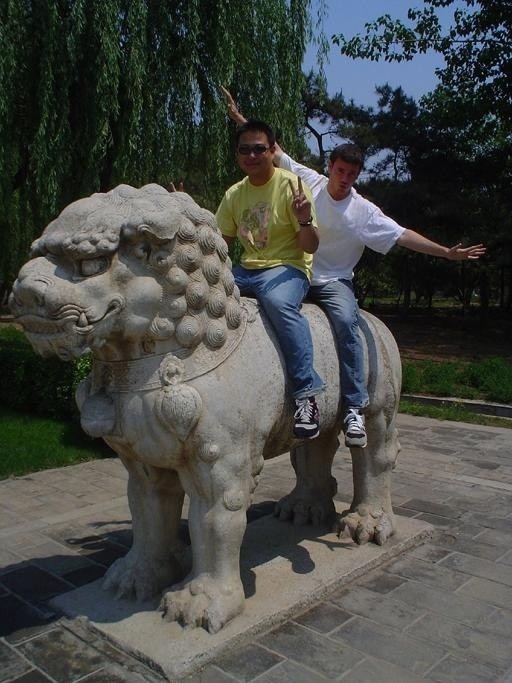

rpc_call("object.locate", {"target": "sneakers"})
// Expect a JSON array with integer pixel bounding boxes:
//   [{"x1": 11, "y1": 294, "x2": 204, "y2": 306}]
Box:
[
  {"x1": 294, "y1": 396, "x2": 320, "y2": 441},
  {"x1": 343, "y1": 407, "x2": 367, "y2": 449}
]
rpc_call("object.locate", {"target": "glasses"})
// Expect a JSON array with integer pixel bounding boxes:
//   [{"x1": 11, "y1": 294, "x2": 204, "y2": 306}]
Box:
[{"x1": 239, "y1": 144, "x2": 270, "y2": 155}]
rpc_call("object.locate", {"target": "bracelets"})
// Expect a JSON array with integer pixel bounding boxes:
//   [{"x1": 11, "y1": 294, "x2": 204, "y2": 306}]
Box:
[{"x1": 297, "y1": 216, "x2": 314, "y2": 226}]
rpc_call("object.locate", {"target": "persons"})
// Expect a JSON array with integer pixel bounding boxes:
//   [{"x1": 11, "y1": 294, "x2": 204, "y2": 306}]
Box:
[
  {"x1": 166, "y1": 118, "x2": 328, "y2": 442},
  {"x1": 214, "y1": 82, "x2": 490, "y2": 451}
]
